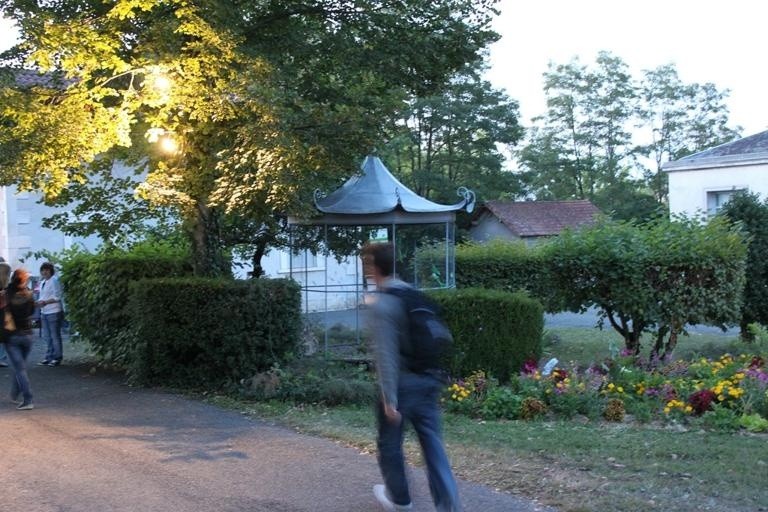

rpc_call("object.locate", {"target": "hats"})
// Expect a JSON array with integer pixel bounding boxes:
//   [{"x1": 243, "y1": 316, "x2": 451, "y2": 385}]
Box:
[{"x1": 12, "y1": 269, "x2": 29, "y2": 284}]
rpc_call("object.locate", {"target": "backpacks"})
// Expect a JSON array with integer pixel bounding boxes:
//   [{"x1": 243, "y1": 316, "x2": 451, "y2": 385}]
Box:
[{"x1": 375, "y1": 285, "x2": 454, "y2": 373}]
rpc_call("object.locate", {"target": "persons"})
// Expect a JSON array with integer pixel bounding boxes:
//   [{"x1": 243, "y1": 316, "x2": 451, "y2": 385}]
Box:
[
  {"x1": 362, "y1": 242, "x2": 460, "y2": 512},
  {"x1": 0, "y1": 257, "x2": 66, "y2": 409}
]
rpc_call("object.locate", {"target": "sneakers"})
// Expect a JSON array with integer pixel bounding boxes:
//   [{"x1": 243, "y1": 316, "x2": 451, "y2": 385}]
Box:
[
  {"x1": 373, "y1": 484, "x2": 412, "y2": 512},
  {"x1": 15, "y1": 401, "x2": 34, "y2": 409},
  {"x1": 36, "y1": 359, "x2": 61, "y2": 366}
]
